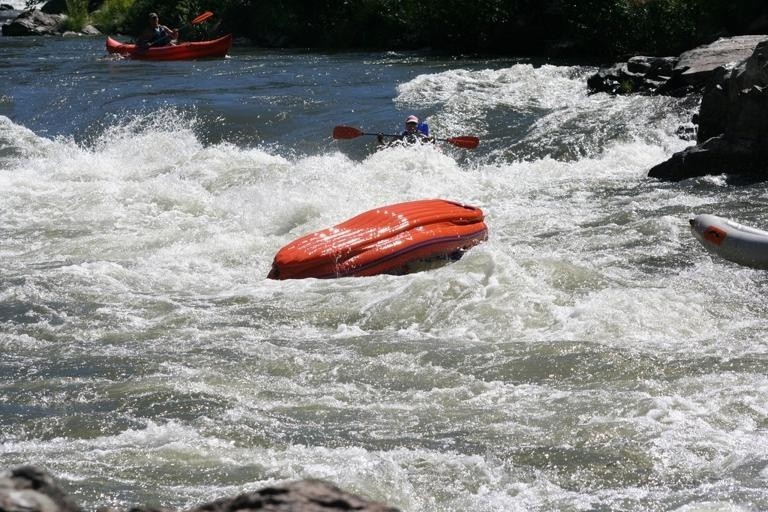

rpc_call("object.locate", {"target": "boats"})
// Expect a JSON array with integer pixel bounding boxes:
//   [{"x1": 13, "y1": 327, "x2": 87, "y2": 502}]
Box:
[
  {"x1": 105, "y1": 34, "x2": 232, "y2": 61},
  {"x1": 270, "y1": 197, "x2": 490, "y2": 280},
  {"x1": 688, "y1": 213, "x2": 767, "y2": 272},
  {"x1": 386, "y1": 137, "x2": 446, "y2": 156}
]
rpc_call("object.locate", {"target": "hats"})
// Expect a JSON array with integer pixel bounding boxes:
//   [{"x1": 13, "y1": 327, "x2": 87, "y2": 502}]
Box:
[
  {"x1": 149, "y1": 12, "x2": 157, "y2": 19},
  {"x1": 406, "y1": 115, "x2": 419, "y2": 124}
]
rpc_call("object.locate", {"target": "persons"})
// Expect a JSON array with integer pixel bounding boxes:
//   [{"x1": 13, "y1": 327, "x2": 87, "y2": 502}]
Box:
[
  {"x1": 138, "y1": 12, "x2": 180, "y2": 48},
  {"x1": 376, "y1": 113, "x2": 434, "y2": 147}
]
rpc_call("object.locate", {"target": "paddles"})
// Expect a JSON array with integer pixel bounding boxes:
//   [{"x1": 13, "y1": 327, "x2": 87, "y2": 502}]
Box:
[
  {"x1": 121, "y1": 12, "x2": 213, "y2": 60},
  {"x1": 333, "y1": 125, "x2": 479, "y2": 149}
]
